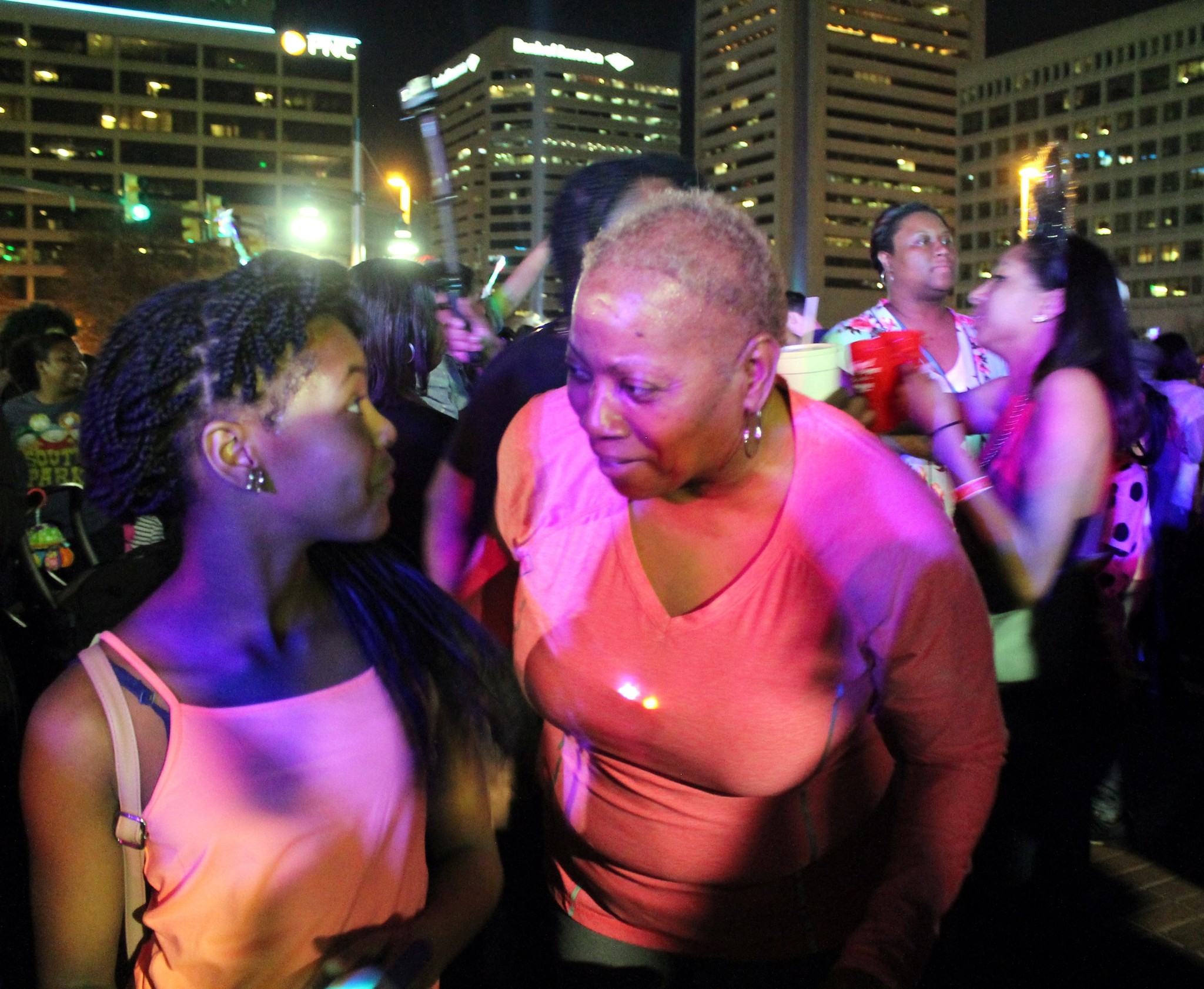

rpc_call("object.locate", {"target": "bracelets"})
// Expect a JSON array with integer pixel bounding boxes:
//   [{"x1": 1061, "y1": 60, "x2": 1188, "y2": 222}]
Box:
[
  {"x1": 929, "y1": 419, "x2": 964, "y2": 438},
  {"x1": 955, "y1": 476, "x2": 996, "y2": 500}
]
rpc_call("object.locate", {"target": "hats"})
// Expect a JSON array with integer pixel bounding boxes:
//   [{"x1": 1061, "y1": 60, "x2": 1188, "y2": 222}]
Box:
[{"x1": 551, "y1": 154, "x2": 704, "y2": 313}]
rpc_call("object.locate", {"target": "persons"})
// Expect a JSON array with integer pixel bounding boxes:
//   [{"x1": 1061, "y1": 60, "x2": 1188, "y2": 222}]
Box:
[{"x1": 0, "y1": 153, "x2": 1204, "y2": 989}]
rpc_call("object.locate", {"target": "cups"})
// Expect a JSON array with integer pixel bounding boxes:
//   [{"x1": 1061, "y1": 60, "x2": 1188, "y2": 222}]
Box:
[
  {"x1": 776, "y1": 342, "x2": 845, "y2": 402},
  {"x1": 850, "y1": 330, "x2": 926, "y2": 435}
]
